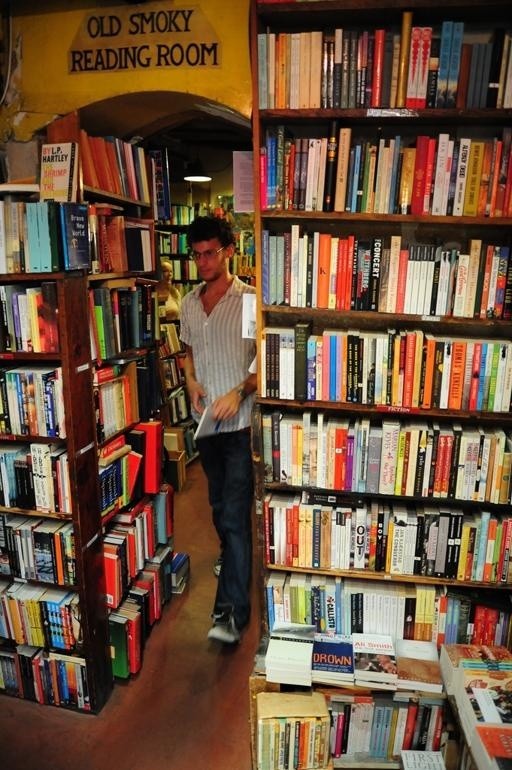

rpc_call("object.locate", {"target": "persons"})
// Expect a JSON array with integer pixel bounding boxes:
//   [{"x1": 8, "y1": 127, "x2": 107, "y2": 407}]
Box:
[{"x1": 178, "y1": 215, "x2": 260, "y2": 649}]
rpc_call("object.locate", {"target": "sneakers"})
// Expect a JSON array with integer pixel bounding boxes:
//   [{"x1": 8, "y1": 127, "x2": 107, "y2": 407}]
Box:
[
  {"x1": 206, "y1": 622, "x2": 241, "y2": 645},
  {"x1": 213, "y1": 554, "x2": 224, "y2": 576}
]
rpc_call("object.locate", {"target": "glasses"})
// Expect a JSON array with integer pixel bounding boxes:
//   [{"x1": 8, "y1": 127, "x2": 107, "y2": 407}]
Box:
[{"x1": 191, "y1": 245, "x2": 226, "y2": 262}]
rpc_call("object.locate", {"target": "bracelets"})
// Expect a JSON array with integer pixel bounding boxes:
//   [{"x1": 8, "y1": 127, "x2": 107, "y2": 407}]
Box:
[{"x1": 233, "y1": 387, "x2": 247, "y2": 400}]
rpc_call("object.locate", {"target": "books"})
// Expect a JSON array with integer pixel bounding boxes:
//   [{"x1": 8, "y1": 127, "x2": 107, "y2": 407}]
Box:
[
  {"x1": 259, "y1": 124, "x2": 512, "y2": 218},
  {"x1": 2, "y1": 129, "x2": 193, "y2": 712},
  {"x1": 228, "y1": 227, "x2": 255, "y2": 288},
  {"x1": 261, "y1": 223, "x2": 512, "y2": 320},
  {"x1": 264, "y1": 490, "x2": 512, "y2": 584},
  {"x1": 250, "y1": 570, "x2": 512, "y2": 770},
  {"x1": 257, "y1": 12, "x2": 512, "y2": 109},
  {"x1": 262, "y1": 411, "x2": 512, "y2": 504},
  {"x1": 262, "y1": 322, "x2": 512, "y2": 413}
]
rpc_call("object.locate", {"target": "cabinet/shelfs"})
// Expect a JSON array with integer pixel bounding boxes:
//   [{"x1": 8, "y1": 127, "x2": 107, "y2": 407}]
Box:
[
  {"x1": 244, "y1": 3, "x2": 512, "y2": 768},
  {"x1": 1, "y1": 109, "x2": 247, "y2": 717}
]
